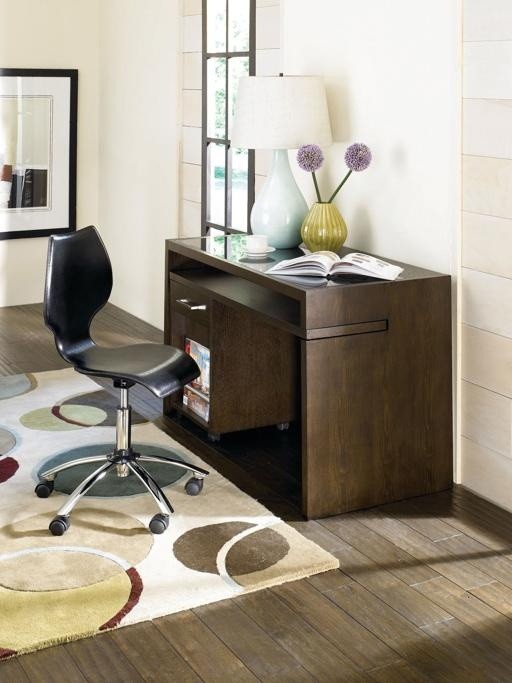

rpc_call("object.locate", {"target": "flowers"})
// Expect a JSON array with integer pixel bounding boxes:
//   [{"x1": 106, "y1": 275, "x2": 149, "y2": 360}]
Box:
[{"x1": 296, "y1": 144, "x2": 373, "y2": 202}]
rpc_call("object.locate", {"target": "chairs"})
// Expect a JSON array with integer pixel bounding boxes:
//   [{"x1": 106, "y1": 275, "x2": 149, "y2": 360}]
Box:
[{"x1": 34, "y1": 227, "x2": 202, "y2": 535}]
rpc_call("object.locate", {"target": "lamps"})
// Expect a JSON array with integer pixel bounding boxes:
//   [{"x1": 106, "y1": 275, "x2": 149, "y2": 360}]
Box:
[{"x1": 230, "y1": 72, "x2": 333, "y2": 249}]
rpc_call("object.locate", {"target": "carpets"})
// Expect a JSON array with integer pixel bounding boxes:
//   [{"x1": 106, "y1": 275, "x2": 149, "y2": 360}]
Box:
[{"x1": 0, "y1": 365, "x2": 340, "y2": 659}]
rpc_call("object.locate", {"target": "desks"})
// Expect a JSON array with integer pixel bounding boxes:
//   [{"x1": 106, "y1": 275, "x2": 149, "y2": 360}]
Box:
[{"x1": 165, "y1": 233, "x2": 459, "y2": 520}]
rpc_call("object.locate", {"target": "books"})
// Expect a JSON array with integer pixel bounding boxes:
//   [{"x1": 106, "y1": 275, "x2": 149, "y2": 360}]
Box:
[{"x1": 268, "y1": 248, "x2": 405, "y2": 281}]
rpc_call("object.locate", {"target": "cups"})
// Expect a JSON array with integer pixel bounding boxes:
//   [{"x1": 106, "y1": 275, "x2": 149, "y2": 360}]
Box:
[{"x1": 240, "y1": 235, "x2": 269, "y2": 251}]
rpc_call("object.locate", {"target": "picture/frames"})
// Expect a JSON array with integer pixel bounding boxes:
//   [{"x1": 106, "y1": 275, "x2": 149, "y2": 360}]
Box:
[{"x1": 0, "y1": 68, "x2": 77, "y2": 241}]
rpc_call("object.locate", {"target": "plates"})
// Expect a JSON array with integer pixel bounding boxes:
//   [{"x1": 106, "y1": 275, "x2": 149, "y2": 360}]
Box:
[{"x1": 240, "y1": 247, "x2": 276, "y2": 257}]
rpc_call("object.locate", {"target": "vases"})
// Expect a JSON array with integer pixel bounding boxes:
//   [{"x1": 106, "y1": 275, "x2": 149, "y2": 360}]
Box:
[{"x1": 300, "y1": 201, "x2": 349, "y2": 253}]
